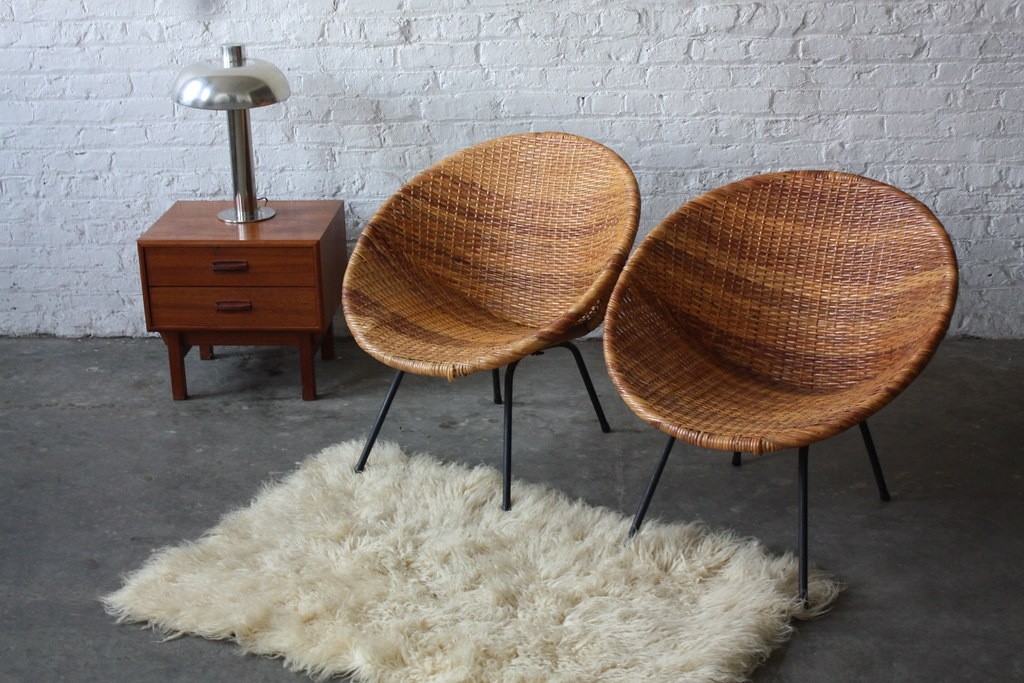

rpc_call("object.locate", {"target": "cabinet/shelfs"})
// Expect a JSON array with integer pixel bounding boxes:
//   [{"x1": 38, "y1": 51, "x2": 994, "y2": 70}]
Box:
[{"x1": 137, "y1": 198, "x2": 349, "y2": 402}]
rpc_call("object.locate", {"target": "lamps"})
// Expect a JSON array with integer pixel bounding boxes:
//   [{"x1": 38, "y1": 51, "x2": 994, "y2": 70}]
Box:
[{"x1": 169, "y1": 41, "x2": 293, "y2": 223}]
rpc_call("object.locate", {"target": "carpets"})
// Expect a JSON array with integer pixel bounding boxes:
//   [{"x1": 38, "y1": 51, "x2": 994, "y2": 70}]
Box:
[{"x1": 105, "y1": 435, "x2": 849, "y2": 683}]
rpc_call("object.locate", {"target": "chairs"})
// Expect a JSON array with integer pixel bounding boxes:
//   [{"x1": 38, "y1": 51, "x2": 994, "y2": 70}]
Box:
[
  {"x1": 344, "y1": 130, "x2": 642, "y2": 517},
  {"x1": 601, "y1": 170, "x2": 959, "y2": 623}
]
